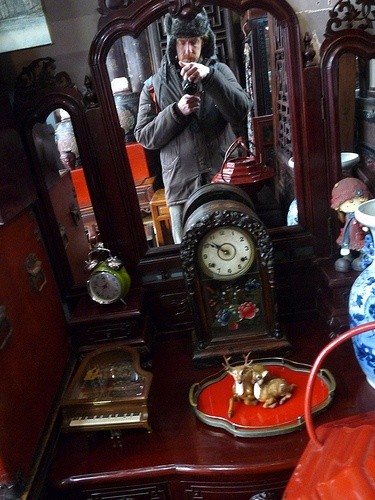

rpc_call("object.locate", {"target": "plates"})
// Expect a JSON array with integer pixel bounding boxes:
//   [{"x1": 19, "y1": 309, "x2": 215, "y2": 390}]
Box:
[{"x1": 188, "y1": 357, "x2": 336, "y2": 437}]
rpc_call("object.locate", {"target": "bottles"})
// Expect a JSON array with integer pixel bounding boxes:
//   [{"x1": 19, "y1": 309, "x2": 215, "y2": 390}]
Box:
[
  {"x1": 288, "y1": 153, "x2": 359, "y2": 225},
  {"x1": 60, "y1": 149, "x2": 77, "y2": 171},
  {"x1": 348, "y1": 199, "x2": 375, "y2": 390}
]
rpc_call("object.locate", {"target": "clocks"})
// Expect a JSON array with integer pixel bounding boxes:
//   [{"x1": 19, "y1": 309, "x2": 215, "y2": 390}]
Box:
[
  {"x1": 180, "y1": 203, "x2": 292, "y2": 361},
  {"x1": 82, "y1": 240, "x2": 129, "y2": 306}
]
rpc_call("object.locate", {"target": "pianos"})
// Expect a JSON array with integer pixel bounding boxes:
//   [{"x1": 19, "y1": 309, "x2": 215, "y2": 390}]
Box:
[{"x1": 58, "y1": 346, "x2": 154, "y2": 437}]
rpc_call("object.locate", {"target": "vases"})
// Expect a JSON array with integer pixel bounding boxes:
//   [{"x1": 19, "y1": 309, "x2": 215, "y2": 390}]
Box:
[{"x1": 348, "y1": 202, "x2": 375, "y2": 388}]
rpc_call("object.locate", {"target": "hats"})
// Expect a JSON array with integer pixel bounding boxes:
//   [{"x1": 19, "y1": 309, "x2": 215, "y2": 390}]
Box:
[{"x1": 164, "y1": 6, "x2": 217, "y2": 65}]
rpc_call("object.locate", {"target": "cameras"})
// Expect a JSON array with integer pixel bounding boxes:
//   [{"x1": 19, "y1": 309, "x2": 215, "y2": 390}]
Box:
[{"x1": 182, "y1": 79, "x2": 200, "y2": 95}]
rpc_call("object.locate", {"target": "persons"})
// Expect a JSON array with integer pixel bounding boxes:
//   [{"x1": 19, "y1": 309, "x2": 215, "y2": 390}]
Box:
[
  {"x1": 133, "y1": 3, "x2": 254, "y2": 245},
  {"x1": 330, "y1": 178, "x2": 375, "y2": 272}
]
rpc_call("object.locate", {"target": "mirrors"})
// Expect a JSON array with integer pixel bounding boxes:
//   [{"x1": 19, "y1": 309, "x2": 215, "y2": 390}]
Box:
[{"x1": 11, "y1": 0, "x2": 375, "y2": 368}]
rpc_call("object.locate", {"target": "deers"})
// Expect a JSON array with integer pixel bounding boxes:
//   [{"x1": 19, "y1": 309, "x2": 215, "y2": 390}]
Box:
[{"x1": 219, "y1": 348, "x2": 297, "y2": 419}]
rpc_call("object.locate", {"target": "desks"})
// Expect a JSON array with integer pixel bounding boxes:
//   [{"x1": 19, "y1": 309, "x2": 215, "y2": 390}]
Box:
[
  {"x1": 149, "y1": 189, "x2": 172, "y2": 246},
  {"x1": 47, "y1": 323, "x2": 375, "y2": 500}
]
